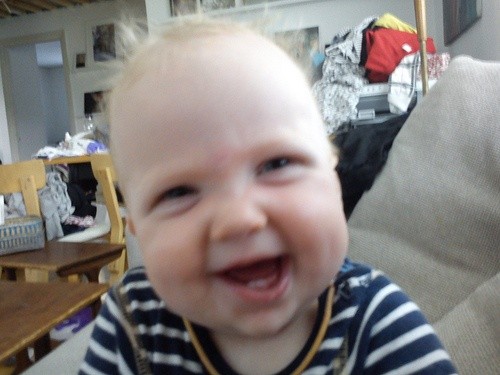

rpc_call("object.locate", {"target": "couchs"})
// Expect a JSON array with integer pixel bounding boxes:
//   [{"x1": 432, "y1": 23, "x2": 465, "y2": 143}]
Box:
[{"x1": 20, "y1": 53, "x2": 500, "y2": 375}]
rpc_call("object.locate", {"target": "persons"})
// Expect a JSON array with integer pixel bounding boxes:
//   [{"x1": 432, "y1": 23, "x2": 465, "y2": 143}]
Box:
[{"x1": 77, "y1": 17, "x2": 460, "y2": 375}]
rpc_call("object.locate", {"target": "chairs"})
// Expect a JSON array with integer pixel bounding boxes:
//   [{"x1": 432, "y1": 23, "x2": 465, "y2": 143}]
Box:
[{"x1": 0, "y1": 148, "x2": 129, "y2": 287}]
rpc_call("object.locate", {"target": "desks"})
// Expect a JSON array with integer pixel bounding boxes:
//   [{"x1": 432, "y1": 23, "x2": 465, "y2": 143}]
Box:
[
  {"x1": 0, "y1": 279, "x2": 112, "y2": 375},
  {"x1": 0, "y1": 237, "x2": 127, "y2": 320}
]
